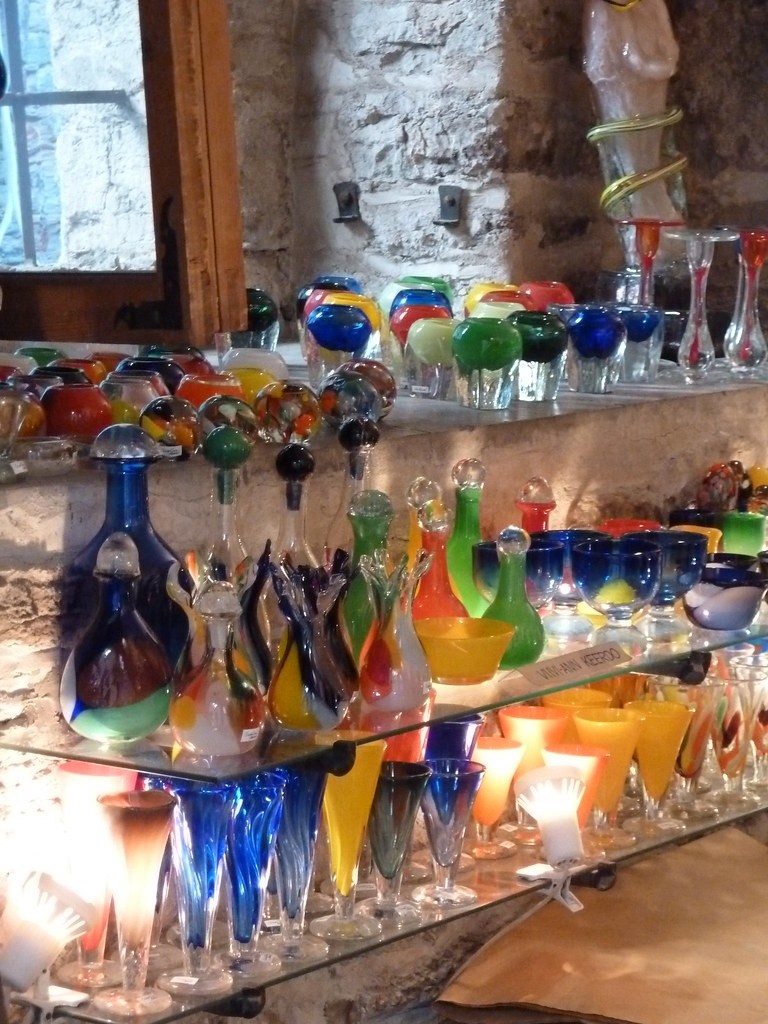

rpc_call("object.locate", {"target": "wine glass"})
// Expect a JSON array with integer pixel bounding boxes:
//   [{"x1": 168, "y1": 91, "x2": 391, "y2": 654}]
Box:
[{"x1": 56, "y1": 531, "x2": 767, "y2": 1011}]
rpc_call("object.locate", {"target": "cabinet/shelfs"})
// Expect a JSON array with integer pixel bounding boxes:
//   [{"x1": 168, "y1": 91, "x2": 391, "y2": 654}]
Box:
[{"x1": 0, "y1": 352, "x2": 768, "y2": 1024}]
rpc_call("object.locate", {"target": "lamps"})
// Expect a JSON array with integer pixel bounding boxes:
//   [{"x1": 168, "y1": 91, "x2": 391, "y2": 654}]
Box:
[{"x1": 509, "y1": 762, "x2": 590, "y2": 915}]
